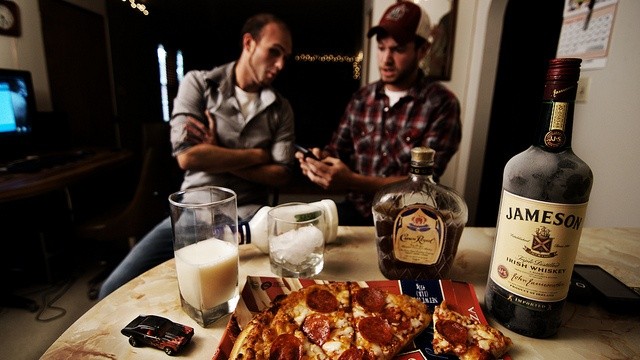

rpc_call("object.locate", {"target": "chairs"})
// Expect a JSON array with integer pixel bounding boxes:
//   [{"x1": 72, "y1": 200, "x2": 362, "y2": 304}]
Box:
[{"x1": 68, "y1": 121, "x2": 185, "y2": 300}]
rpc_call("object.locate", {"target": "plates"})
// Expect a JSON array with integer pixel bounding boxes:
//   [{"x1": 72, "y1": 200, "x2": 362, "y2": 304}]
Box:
[{"x1": 210, "y1": 276, "x2": 512, "y2": 360}]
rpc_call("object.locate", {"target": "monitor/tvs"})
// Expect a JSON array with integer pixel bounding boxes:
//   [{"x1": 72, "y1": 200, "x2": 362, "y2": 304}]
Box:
[{"x1": 0, "y1": 69, "x2": 39, "y2": 141}]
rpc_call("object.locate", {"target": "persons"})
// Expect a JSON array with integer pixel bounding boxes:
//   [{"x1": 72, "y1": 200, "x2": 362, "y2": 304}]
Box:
[
  {"x1": 293, "y1": 1, "x2": 461, "y2": 224},
  {"x1": 101, "y1": 15, "x2": 295, "y2": 301}
]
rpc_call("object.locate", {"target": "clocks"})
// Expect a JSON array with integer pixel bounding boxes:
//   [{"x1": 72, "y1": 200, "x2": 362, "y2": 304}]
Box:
[{"x1": 1, "y1": 2, "x2": 21, "y2": 39}]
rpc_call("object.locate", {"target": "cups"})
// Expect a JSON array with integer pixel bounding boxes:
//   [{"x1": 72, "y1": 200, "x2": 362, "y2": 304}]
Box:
[
  {"x1": 168, "y1": 185, "x2": 240, "y2": 328},
  {"x1": 267, "y1": 202, "x2": 325, "y2": 279}
]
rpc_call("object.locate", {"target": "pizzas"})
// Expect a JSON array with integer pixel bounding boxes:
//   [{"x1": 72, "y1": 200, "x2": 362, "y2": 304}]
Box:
[
  {"x1": 227, "y1": 280, "x2": 431, "y2": 360},
  {"x1": 432, "y1": 301, "x2": 514, "y2": 360}
]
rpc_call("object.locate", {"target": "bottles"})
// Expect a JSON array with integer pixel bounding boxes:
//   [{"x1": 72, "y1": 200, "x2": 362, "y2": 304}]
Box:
[
  {"x1": 483, "y1": 58, "x2": 594, "y2": 339},
  {"x1": 212, "y1": 199, "x2": 338, "y2": 254},
  {"x1": 371, "y1": 146, "x2": 469, "y2": 280}
]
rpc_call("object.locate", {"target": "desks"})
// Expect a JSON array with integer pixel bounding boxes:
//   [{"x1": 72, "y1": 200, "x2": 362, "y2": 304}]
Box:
[{"x1": 2, "y1": 138, "x2": 132, "y2": 201}]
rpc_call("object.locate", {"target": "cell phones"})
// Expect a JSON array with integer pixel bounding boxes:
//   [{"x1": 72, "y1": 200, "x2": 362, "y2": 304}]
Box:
[{"x1": 294, "y1": 142, "x2": 312, "y2": 157}]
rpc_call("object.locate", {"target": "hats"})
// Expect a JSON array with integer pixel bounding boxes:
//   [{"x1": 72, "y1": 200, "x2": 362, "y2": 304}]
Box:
[{"x1": 367, "y1": 1, "x2": 432, "y2": 47}]
